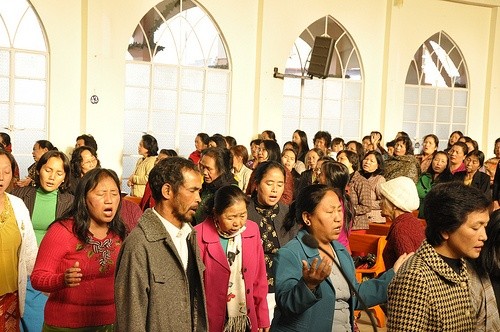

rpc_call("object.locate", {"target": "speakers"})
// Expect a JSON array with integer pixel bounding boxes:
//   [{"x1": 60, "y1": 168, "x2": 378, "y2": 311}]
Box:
[{"x1": 307, "y1": 35, "x2": 336, "y2": 78}]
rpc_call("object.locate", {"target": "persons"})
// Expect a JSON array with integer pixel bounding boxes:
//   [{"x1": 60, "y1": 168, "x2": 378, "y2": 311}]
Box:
[{"x1": 0, "y1": 130, "x2": 500, "y2": 332}]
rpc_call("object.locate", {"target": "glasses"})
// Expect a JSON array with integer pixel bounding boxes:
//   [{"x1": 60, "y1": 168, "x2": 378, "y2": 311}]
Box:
[{"x1": 82, "y1": 157, "x2": 97, "y2": 166}]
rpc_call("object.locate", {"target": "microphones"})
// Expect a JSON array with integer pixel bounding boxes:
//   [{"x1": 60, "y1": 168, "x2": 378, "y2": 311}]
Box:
[{"x1": 302, "y1": 234, "x2": 378, "y2": 332}]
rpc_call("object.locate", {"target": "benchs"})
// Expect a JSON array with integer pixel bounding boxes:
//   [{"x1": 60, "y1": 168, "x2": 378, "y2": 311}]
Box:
[{"x1": 348, "y1": 224, "x2": 391, "y2": 332}]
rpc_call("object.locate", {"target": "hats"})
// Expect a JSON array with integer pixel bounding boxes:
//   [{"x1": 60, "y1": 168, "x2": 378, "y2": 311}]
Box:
[{"x1": 379, "y1": 175, "x2": 420, "y2": 213}]
